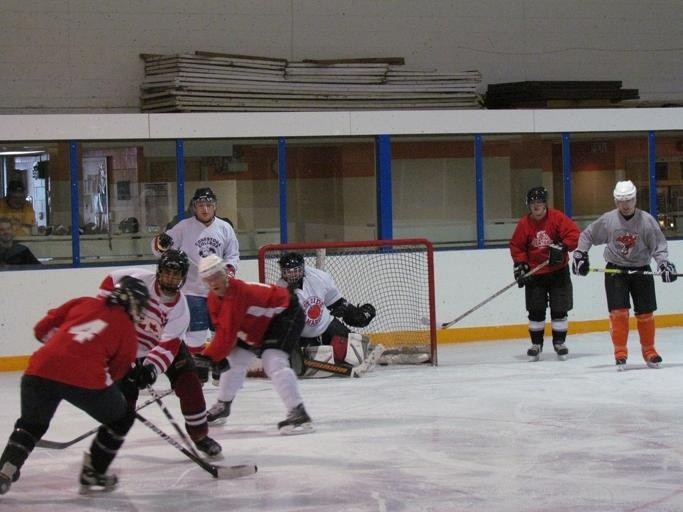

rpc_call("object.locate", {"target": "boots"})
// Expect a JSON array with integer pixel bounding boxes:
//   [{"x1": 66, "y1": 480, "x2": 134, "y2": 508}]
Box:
[
  {"x1": 0, "y1": 459, "x2": 20, "y2": 493},
  {"x1": 79, "y1": 452, "x2": 118, "y2": 486}
]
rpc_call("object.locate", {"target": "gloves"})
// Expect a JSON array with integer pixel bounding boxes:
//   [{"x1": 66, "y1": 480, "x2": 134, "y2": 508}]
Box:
[
  {"x1": 548, "y1": 242, "x2": 567, "y2": 266},
  {"x1": 572, "y1": 249, "x2": 590, "y2": 276},
  {"x1": 659, "y1": 262, "x2": 677, "y2": 283},
  {"x1": 158, "y1": 232, "x2": 175, "y2": 249},
  {"x1": 514, "y1": 262, "x2": 533, "y2": 288},
  {"x1": 191, "y1": 352, "x2": 212, "y2": 384},
  {"x1": 119, "y1": 356, "x2": 157, "y2": 402},
  {"x1": 212, "y1": 358, "x2": 231, "y2": 380}
]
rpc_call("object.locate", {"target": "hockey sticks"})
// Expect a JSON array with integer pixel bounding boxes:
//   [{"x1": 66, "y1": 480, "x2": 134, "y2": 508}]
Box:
[
  {"x1": 303, "y1": 344, "x2": 386, "y2": 378},
  {"x1": 135, "y1": 383, "x2": 257, "y2": 479},
  {"x1": 421, "y1": 261, "x2": 548, "y2": 329},
  {"x1": 36, "y1": 387, "x2": 174, "y2": 450}
]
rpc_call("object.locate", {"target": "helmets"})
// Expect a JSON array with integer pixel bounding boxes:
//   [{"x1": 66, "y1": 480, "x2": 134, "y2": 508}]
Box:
[
  {"x1": 156, "y1": 248, "x2": 189, "y2": 292},
  {"x1": 121, "y1": 217, "x2": 138, "y2": 233},
  {"x1": 192, "y1": 187, "x2": 217, "y2": 209},
  {"x1": 613, "y1": 180, "x2": 636, "y2": 209},
  {"x1": 106, "y1": 276, "x2": 150, "y2": 323},
  {"x1": 198, "y1": 254, "x2": 224, "y2": 279},
  {"x1": 279, "y1": 252, "x2": 305, "y2": 283},
  {"x1": 525, "y1": 187, "x2": 548, "y2": 205}
]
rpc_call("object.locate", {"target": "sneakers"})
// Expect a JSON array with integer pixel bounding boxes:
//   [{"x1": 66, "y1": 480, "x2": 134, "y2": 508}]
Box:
[
  {"x1": 278, "y1": 403, "x2": 312, "y2": 429},
  {"x1": 527, "y1": 344, "x2": 542, "y2": 356},
  {"x1": 615, "y1": 349, "x2": 627, "y2": 364},
  {"x1": 553, "y1": 343, "x2": 569, "y2": 356},
  {"x1": 206, "y1": 400, "x2": 232, "y2": 423},
  {"x1": 642, "y1": 346, "x2": 662, "y2": 363},
  {"x1": 196, "y1": 435, "x2": 222, "y2": 456}
]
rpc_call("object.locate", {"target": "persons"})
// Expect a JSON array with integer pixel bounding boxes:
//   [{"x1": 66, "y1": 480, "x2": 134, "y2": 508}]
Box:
[
  {"x1": 151, "y1": 187, "x2": 240, "y2": 390},
  {"x1": 99, "y1": 249, "x2": 223, "y2": 455},
  {"x1": 0, "y1": 180, "x2": 36, "y2": 225},
  {"x1": 0, "y1": 276, "x2": 150, "y2": 486},
  {"x1": 276, "y1": 253, "x2": 357, "y2": 369},
  {"x1": 572, "y1": 180, "x2": 677, "y2": 366},
  {"x1": 0, "y1": 218, "x2": 41, "y2": 264},
  {"x1": 510, "y1": 187, "x2": 580, "y2": 357},
  {"x1": 198, "y1": 254, "x2": 312, "y2": 428}
]
